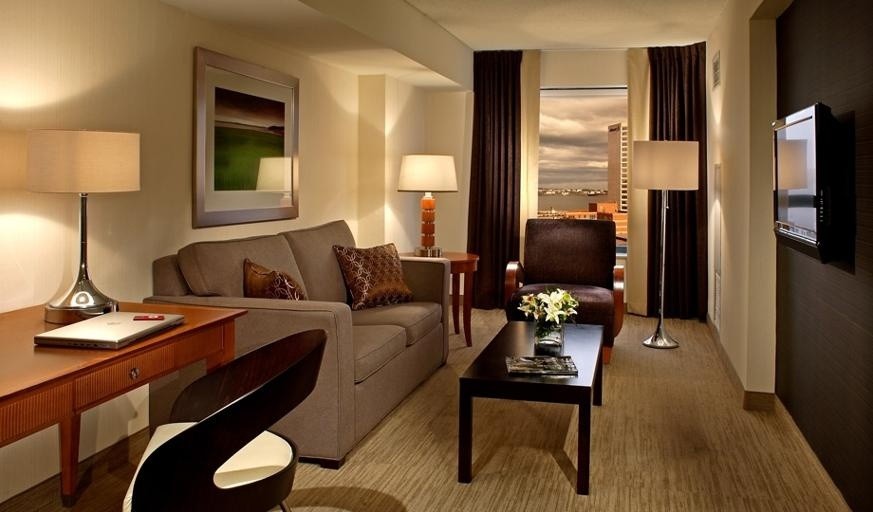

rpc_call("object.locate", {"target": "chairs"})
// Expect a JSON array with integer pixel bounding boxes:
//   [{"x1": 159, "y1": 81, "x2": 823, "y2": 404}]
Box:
[
  {"x1": 501, "y1": 217, "x2": 625, "y2": 366},
  {"x1": 116, "y1": 323, "x2": 331, "y2": 512}
]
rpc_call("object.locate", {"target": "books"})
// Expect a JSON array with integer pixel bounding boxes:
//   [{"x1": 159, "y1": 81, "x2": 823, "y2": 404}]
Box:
[{"x1": 503, "y1": 355, "x2": 578, "y2": 375}]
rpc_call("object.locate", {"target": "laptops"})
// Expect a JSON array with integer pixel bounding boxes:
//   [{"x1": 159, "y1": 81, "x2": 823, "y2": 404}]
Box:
[{"x1": 34, "y1": 311, "x2": 184, "y2": 350}]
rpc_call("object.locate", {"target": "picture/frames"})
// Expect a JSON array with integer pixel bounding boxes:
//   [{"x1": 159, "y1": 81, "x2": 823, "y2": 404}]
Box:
[{"x1": 189, "y1": 43, "x2": 300, "y2": 230}]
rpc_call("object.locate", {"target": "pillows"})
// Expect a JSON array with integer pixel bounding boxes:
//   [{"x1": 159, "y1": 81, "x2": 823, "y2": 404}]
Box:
[
  {"x1": 333, "y1": 242, "x2": 416, "y2": 312},
  {"x1": 241, "y1": 257, "x2": 307, "y2": 302}
]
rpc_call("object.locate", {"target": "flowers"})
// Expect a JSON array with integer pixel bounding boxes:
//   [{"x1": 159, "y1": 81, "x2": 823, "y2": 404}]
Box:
[{"x1": 518, "y1": 285, "x2": 579, "y2": 340}]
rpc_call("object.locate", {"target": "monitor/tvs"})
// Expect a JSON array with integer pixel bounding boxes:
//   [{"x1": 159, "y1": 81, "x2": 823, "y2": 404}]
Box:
[{"x1": 769, "y1": 103, "x2": 832, "y2": 265}]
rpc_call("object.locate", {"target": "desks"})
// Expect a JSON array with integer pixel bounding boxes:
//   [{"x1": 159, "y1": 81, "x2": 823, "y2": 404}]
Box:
[
  {"x1": 457, "y1": 319, "x2": 606, "y2": 499},
  {"x1": 0, "y1": 298, "x2": 247, "y2": 512},
  {"x1": 394, "y1": 250, "x2": 480, "y2": 348}
]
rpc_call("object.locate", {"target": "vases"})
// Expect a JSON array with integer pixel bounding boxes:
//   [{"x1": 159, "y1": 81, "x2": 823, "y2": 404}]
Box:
[{"x1": 533, "y1": 316, "x2": 564, "y2": 358}]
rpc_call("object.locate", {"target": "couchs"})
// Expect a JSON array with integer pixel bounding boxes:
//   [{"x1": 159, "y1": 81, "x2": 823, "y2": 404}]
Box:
[{"x1": 142, "y1": 218, "x2": 450, "y2": 471}]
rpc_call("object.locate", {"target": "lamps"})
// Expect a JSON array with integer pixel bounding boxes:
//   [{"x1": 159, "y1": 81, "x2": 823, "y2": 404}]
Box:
[
  {"x1": 255, "y1": 156, "x2": 298, "y2": 208},
  {"x1": 22, "y1": 128, "x2": 142, "y2": 326},
  {"x1": 627, "y1": 138, "x2": 700, "y2": 350},
  {"x1": 395, "y1": 152, "x2": 458, "y2": 257},
  {"x1": 777, "y1": 140, "x2": 809, "y2": 191}
]
